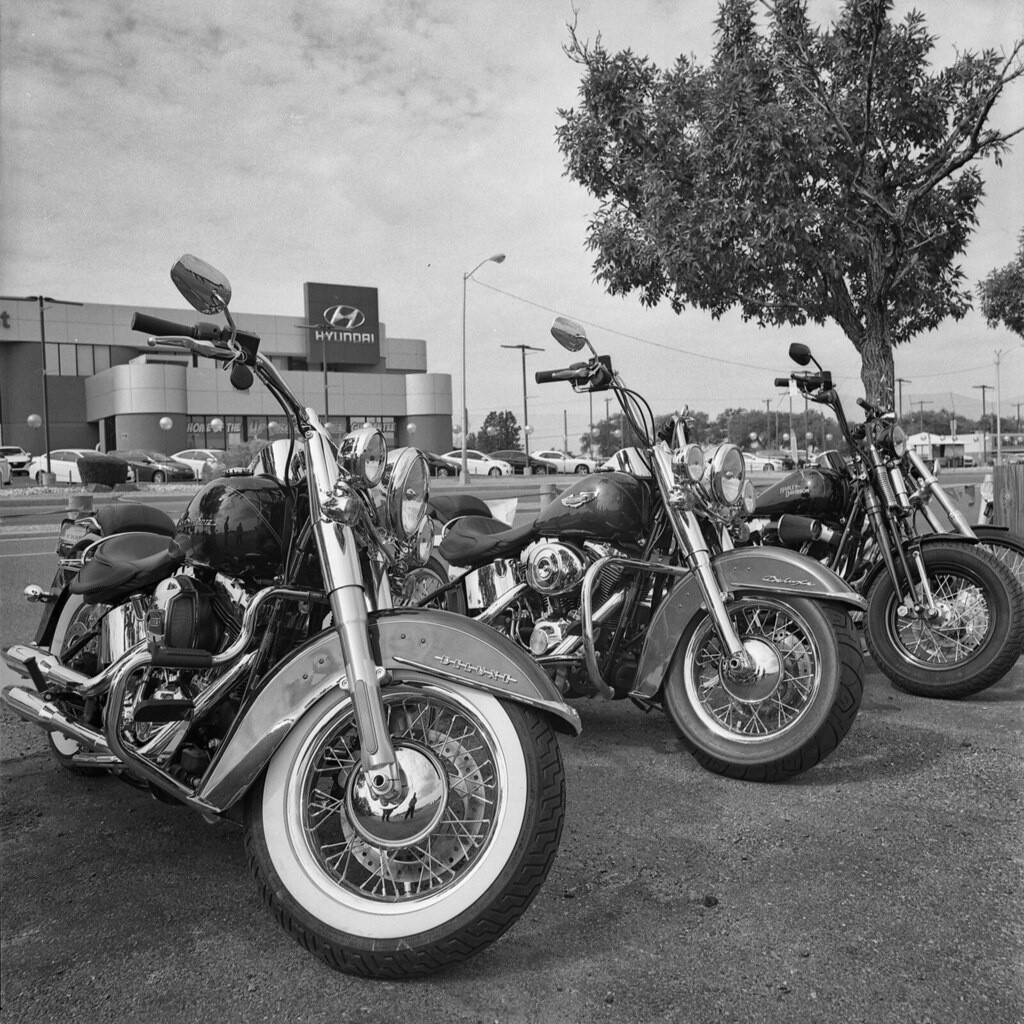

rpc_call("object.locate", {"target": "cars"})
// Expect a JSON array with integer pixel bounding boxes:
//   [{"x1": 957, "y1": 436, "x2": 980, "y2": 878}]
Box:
[
  {"x1": 488, "y1": 450, "x2": 558, "y2": 476},
  {"x1": 31, "y1": 448, "x2": 136, "y2": 485},
  {"x1": 171, "y1": 449, "x2": 239, "y2": 481},
  {"x1": 0, "y1": 446, "x2": 33, "y2": 476},
  {"x1": 0, "y1": 453, "x2": 13, "y2": 485},
  {"x1": 741, "y1": 452, "x2": 784, "y2": 474},
  {"x1": 530, "y1": 450, "x2": 596, "y2": 475},
  {"x1": 107, "y1": 450, "x2": 195, "y2": 484},
  {"x1": 442, "y1": 451, "x2": 513, "y2": 478}
]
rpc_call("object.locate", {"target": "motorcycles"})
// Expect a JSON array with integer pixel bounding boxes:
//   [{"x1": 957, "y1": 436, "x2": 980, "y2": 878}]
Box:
[
  {"x1": 11, "y1": 253, "x2": 587, "y2": 978},
  {"x1": 401, "y1": 317, "x2": 874, "y2": 787},
  {"x1": 622, "y1": 346, "x2": 1024, "y2": 699}
]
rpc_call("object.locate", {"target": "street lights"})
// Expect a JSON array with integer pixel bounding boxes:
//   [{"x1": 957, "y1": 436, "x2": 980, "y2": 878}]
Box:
[{"x1": 459, "y1": 253, "x2": 507, "y2": 484}]
